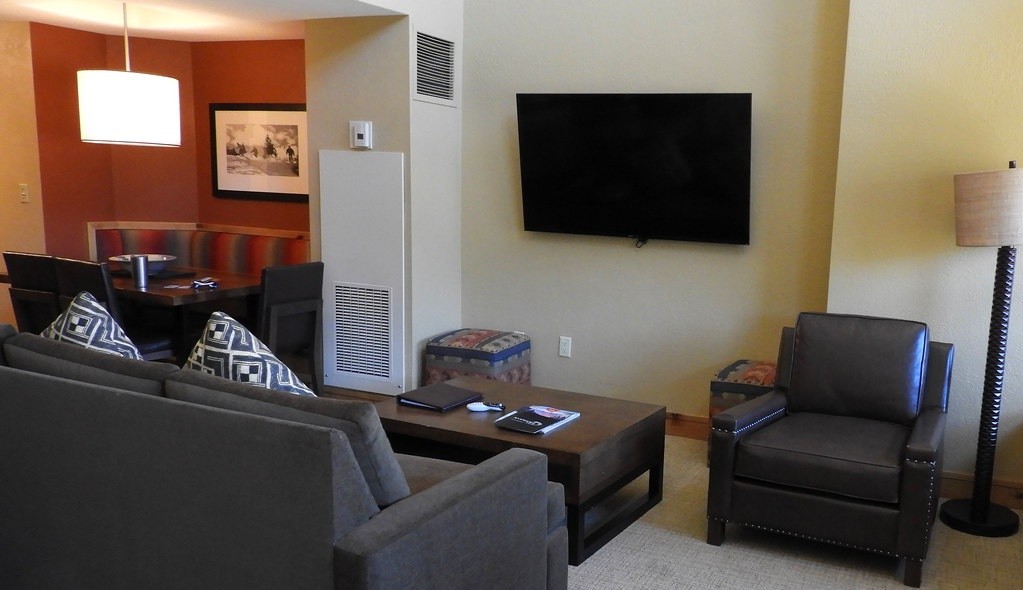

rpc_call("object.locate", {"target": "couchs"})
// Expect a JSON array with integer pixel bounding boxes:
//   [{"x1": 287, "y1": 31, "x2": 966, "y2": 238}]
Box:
[{"x1": 0, "y1": 326, "x2": 571, "y2": 589}]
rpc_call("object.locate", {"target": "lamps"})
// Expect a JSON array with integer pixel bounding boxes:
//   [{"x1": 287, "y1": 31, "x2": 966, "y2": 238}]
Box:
[
  {"x1": 78, "y1": 4, "x2": 180, "y2": 147},
  {"x1": 939, "y1": 160, "x2": 1022, "y2": 538}
]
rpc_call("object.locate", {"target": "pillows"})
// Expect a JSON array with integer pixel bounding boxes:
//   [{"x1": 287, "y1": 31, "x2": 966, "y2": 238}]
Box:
[
  {"x1": 175, "y1": 309, "x2": 322, "y2": 401},
  {"x1": 38, "y1": 293, "x2": 151, "y2": 362}
]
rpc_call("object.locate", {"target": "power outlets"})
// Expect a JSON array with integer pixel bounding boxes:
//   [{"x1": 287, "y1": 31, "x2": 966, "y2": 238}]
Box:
[{"x1": 558, "y1": 335, "x2": 572, "y2": 357}]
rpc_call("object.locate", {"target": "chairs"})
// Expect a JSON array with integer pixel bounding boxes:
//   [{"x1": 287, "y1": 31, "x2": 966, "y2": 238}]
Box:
[
  {"x1": 707, "y1": 312, "x2": 954, "y2": 588},
  {"x1": 183, "y1": 261, "x2": 323, "y2": 395},
  {"x1": 53, "y1": 256, "x2": 176, "y2": 364},
  {"x1": 2, "y1": 251, "x2": 56, "y2": 335}
]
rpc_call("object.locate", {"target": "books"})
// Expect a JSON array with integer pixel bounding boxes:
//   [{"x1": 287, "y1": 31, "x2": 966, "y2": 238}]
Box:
[{"x1": 493, "y1": 403, "x2": 581, "y2": 434}]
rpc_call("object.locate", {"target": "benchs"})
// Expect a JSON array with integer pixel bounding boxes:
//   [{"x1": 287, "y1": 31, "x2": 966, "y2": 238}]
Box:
[{"x1": 87, "y1": 222, "x2": 311, "y2": 279}]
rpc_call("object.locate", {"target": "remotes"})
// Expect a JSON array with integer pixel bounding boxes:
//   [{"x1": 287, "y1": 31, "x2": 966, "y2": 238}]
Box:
[{"x1": 467, "y1": 401, "x2": 505, "y2": 412}]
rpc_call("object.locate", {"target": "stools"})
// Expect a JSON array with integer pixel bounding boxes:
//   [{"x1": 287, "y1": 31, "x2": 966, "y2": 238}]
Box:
[
  {"x1": 706, "y1": 358, "x2": 778, "y2": 470},
  {"x1": 420, "y1": 330, "x2": 537, "y2": 387}
]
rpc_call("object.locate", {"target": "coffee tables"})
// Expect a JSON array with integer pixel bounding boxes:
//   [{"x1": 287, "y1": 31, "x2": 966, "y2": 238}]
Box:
[{"x1": 373, "y1": 376, "x2": 667, "y2": 566}]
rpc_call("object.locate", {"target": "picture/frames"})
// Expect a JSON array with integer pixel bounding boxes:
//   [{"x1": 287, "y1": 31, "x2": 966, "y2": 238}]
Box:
[{"x1": 210, "y1": 102, "x2": 308, "y2": 203}]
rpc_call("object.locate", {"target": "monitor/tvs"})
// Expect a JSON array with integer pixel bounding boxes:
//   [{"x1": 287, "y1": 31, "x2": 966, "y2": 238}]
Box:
[{"x1": 516, "y1": 92, "x2": 752, "y2": 245}]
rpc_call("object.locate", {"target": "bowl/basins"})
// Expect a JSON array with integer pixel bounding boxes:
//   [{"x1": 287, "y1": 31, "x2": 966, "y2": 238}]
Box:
[{"x1": 108, "y1": 253, "x2": 177, "y2": 274}]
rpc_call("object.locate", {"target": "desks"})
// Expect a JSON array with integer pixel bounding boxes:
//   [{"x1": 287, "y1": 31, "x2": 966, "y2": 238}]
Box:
[{"x1": 113, "y1": 271, "x2": 260, "y2": 367}]
